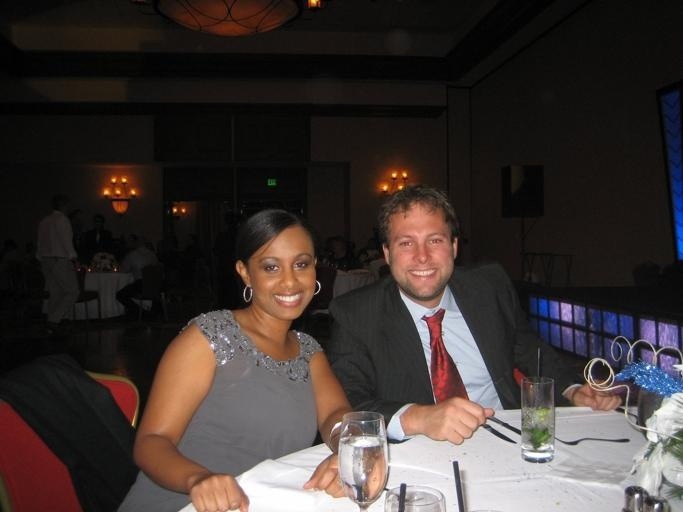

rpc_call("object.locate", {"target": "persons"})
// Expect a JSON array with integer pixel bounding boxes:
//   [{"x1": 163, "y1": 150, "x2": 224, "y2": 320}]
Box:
[
  {"x1": 87, "y1": 214, "x2": 113, "y2": 254},
  {"x1": 115, "y1": 209, "x2": 387, "y2": 511},
  {"x1": 71, "y1": 208, "x2": 103, "y2": 264},
  {"x1": 35, "y1": 194, "x2": 80, "y2": 324},
  {"x1": 328, "y1": 184, "x2": 623, "y2": 446},
  {"x1": 21, "y1": 240, "x2": 35, "y2": 263},
  {"x1": 213, "y1": 212, "x2": 244, "y2": 307},
  {"x1": 115, "y1": 234, "x2": 153, "y2": 321},
  {"x1": 328, "y1": 236, "x2": 351, "y2": 265},
  {"x1": 1, "y1": 238, "x2": 18, "y2": 266}
]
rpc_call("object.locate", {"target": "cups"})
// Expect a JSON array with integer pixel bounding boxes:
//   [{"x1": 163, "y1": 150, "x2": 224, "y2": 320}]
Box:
[{"x1": 520, "y1": 376, "x2": 555, "y2": 464}]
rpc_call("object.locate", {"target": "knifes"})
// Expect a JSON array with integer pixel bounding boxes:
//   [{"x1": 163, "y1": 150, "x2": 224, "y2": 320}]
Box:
[
  {"x1": 487, "y1": 411, "x2": 522, "y2": 436},
  {"x1": 483, "y1": 424, "x2": 517, "y2": 445}
]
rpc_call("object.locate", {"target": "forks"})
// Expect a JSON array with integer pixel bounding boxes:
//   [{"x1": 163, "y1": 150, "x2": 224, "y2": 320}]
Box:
[{"x1": 555, "y1": 437, "x2": 631, "y2": 446}]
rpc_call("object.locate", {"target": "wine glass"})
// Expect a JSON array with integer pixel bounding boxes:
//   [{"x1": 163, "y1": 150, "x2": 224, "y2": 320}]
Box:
[
  {"x1": 338, "y1": 410, "x2": 390, "y2": 512},
  {"x1": 385, "y1": 485, "x2": 446, "y2": 512}
]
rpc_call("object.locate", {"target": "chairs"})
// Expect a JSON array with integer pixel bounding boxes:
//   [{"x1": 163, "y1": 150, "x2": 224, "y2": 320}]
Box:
[
  {"x1": 303, "y1": 264, "x2": 336, "y2": 338},
  {"x1": 125, "y1": 269, "x2": 171, "y2": 323},
  {"x1": 73, "y1": 267, "x2": 102, "y2": 323},
  {"x1": 21, "y1": 265, "x2": 51, "y2": 324},
  {"x1": 0, "y1": 371, "x2": 140, "y2": 512}
]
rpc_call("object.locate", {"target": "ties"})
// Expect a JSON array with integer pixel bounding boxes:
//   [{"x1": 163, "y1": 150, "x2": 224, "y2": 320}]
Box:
[{"x1": 420, "y1": 308, "x2": 470, "y2": 405}]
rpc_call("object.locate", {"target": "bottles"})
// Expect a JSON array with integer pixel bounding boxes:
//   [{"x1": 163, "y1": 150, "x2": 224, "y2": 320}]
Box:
[
  {"x1": 620, "y1": 484, "x2": 648, "y2": 512},
  {"x1": 646, "y1": 495, "x2": 671, "y2": 512}
]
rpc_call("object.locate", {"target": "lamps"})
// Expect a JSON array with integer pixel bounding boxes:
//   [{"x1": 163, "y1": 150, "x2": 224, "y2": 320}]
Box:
[
  {"x1": 102, "y1": 175, "x2": 137, "y2": 219},
  {"x1": 379, "y1": 170, "x2": 415, "y2": 199},
  {"x1": 131, "y1": 0, "x2": 325, "y2": 40}
]
